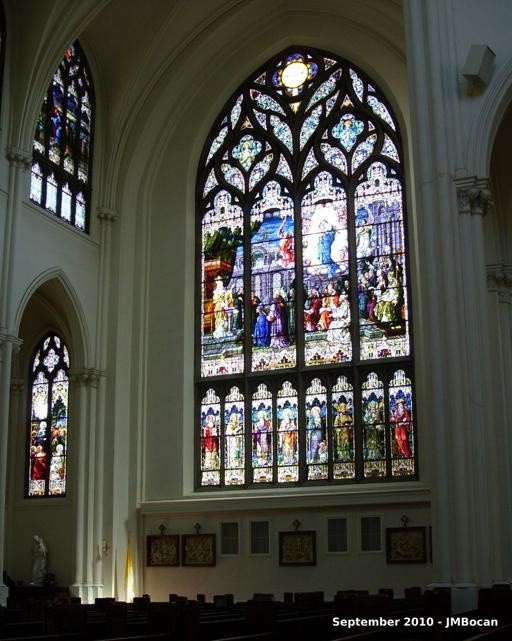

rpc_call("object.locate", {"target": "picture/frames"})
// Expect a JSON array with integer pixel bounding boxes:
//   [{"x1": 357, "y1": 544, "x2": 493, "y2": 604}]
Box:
[
  {"x1": 146, "y1": 532, "x2": 217, "y2": 568},
  {"x1": 278, "y1": 530, "x2": 317, "y2": 567},
  {"x1": 384, "y1": 527, "x2": 427, "y2": 564}
]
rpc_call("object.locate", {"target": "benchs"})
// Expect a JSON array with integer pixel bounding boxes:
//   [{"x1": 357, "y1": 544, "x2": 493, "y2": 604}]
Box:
[{"x1": 3, "y1": 589, "x2": 511, "y2": 640}]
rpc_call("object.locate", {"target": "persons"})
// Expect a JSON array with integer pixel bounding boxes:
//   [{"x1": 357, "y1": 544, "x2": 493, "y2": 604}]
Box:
[
  {"x1": 211, "y1": 246, "x2": 404, "y2": 347},
  {"x1": 32, "y1": 424, "x2": 67, "y2": 480},
  {"x1": 203, "y1": 398, "x2": 412, "y2": 467}
]
